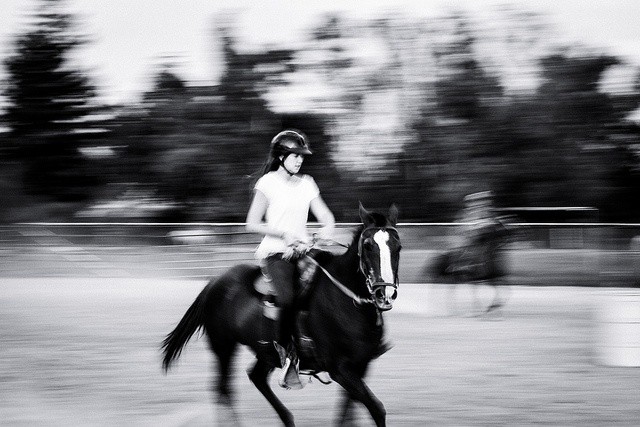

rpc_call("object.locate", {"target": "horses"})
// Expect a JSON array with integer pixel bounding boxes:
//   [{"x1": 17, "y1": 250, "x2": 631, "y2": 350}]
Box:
[{"x1": 159, "y1": 200, "x2": 402, "y2": 427}]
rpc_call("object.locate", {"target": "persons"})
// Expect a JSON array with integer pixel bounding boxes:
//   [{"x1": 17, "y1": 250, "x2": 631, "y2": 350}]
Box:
[{"x1": 244, "y1": 130, "x2": 335, "y2": 391}]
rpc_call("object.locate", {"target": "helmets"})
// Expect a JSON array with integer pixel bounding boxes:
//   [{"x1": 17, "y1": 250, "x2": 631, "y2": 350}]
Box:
[{"x1": 268, "y1": 130, "x2": 313, "y2": 156}]
